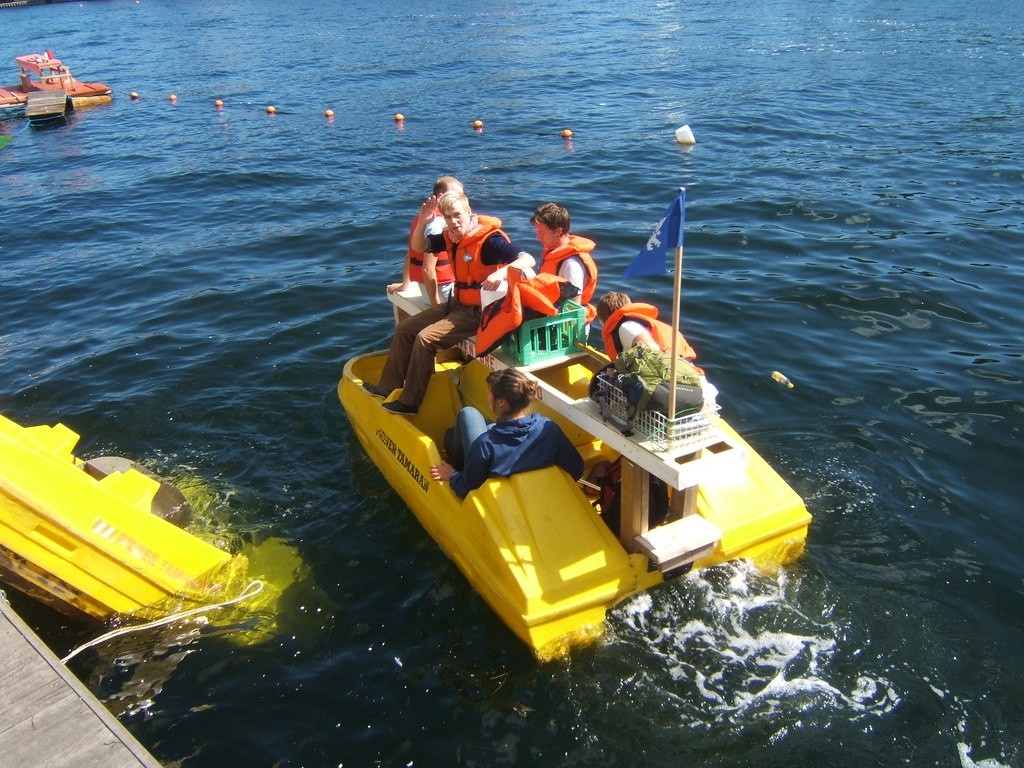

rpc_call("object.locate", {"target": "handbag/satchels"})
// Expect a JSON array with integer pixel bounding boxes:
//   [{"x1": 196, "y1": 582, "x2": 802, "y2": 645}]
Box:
[{"x1": 592, "y1": 455, "x2": 670, "y2": 535}]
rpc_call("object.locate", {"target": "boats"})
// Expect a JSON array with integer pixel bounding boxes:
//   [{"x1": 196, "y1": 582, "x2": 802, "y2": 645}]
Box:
[
  {"x1": 0, "y1": 413, "x2": 310, "y2": 650},
  {"x1": 0, "y1": 48, "x2": 112, "y2": 119},
  {"x1": 335, "y1": 280, "x2": 815, "y2": 670}
]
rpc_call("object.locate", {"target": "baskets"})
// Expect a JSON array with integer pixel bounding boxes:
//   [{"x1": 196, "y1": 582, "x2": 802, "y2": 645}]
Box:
[
  {"x1": 501, "y1": 298, "x2": 586, "y2": 366},
  {"x1": 597, "y1": 373, "x2": 722, "y2": 453}
]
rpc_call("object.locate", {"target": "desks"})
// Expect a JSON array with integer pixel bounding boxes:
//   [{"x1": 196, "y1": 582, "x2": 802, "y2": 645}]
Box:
[{"x1": 16, "y1": 54, "x2": 66, "y2": 89}]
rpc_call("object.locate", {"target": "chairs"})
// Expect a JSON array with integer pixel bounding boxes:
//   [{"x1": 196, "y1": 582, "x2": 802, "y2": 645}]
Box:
[{"x1": 45, "y1": 49, "x2": 69, "y2": 81}]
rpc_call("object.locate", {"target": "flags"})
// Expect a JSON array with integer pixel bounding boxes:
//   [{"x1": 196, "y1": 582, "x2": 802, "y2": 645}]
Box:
[{"x1": 622, "y1": 189, "x2": 685, "y2": 280}]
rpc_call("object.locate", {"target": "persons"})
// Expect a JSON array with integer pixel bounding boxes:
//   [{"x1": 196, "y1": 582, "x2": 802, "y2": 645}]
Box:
[
  {"x1": 361, "y1": 190, "x2": 536, "y2": 414},
  {"x1": 386, "y1": 175, "x2": 464, "y2": 306},
  {"x1": 530, "y1": 202, "x2": 597, "y2": 341},
  {"x1": 596, "y1": 291, "x2": 696, "y2": 370},
  {"x1": 429, "y1": 367, "x2": 584, "y2": 500}
]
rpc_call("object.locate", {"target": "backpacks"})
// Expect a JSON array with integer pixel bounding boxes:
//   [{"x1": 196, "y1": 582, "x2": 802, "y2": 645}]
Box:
[{"x1": 591, "y1": 344, "x2": 704, "y2": 437}]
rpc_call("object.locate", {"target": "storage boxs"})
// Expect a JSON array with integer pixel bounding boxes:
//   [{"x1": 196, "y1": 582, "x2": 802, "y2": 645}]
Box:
[
  {"x1": 630, "y1": 403, "x2": 722, "y2": 452},
  {"x1": 596, "y1": 373, "x2": 629, "y2": 426},
  {"x1": 501, "y1": 298, "x2": 587, "y2": 366}
]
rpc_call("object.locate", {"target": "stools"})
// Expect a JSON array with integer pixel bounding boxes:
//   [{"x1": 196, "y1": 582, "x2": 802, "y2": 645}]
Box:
[{"x1": 19, "y1": 73, "x2": 33, "y2": 89}]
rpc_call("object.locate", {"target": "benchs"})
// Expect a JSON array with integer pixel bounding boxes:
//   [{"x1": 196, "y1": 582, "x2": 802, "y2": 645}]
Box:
[{"x1": 40, "y1": 75, "x2": 74, "y2": 91}]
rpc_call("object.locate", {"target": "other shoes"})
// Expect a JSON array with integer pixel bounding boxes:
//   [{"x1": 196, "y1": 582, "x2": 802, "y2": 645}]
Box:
[
  {"x1": 361, "y1": 381, "x2": 392, "y2": 399},
  {"x1": 381, "y1": 400, "x2": 419, "y2": 416},
  {"x1": 444, "y1": 428, "x2": 455, "y2": 458}
]
